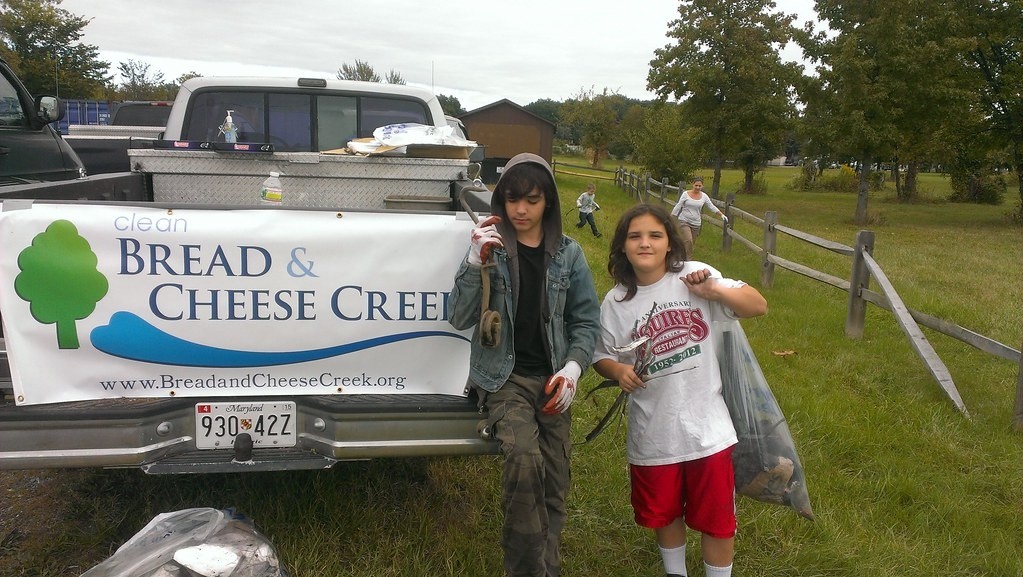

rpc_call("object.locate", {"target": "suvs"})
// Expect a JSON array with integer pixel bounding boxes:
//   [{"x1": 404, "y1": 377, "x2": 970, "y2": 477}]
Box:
[{"x1": 0, "y1": 59, "x2": 89, "y2": 181}]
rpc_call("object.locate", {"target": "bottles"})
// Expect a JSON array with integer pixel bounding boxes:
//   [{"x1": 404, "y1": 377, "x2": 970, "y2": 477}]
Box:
[
  {"x1": 261, "y1": 172, "x2": 282, "y2": 206},
  {"x1": 225, "y1": 116, "x2": 237, "y2": 143}
]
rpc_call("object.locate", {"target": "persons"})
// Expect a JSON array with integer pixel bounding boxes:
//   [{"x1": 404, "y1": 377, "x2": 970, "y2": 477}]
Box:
[
  {"x1": 447, "y1": 152, "x2": 601, "y2": 577},
  {"x1": 671, "y1": 179, "x2": 729, "y2": 262},
  {"x1": 575, "y1": 184, "x2": 603, "y2": 238},
  {"x1": 591, "y1": 205, "x2": 768, "y2": 577}
]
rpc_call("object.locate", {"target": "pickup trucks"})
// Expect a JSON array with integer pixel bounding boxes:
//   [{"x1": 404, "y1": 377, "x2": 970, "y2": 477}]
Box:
[
  {"x1": 1, "y1": 79, "x2": 515, "y2": 471},
  {"x1": 67, "y1": 100, "x2": 176, "y2": 138}
]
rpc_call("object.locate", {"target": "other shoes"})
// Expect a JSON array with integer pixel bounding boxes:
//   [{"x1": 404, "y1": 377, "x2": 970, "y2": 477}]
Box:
[{"x1": 596, "y1": 233, "x2": 601, "y2": 238}]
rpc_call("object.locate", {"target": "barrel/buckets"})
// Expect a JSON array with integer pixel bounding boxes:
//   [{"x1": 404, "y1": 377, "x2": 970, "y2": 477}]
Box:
[
  {"x1": 469, "y1": 146, "x2": 485, "y2": 162},
  {"x1": 383, "y1": 195, "x2": 451, "y2": 211}
]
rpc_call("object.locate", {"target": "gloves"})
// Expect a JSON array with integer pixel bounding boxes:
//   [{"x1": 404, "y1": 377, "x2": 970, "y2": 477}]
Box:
[
  {"x1": 541, "y1": 360, "x2": 582, "y2": 417},
  {"x1": 721, "y1": 214, "x2": 728, "y2": 223},
  {"x1": 466, "y1": 216, "x2": 504, "y2": 267}
]
